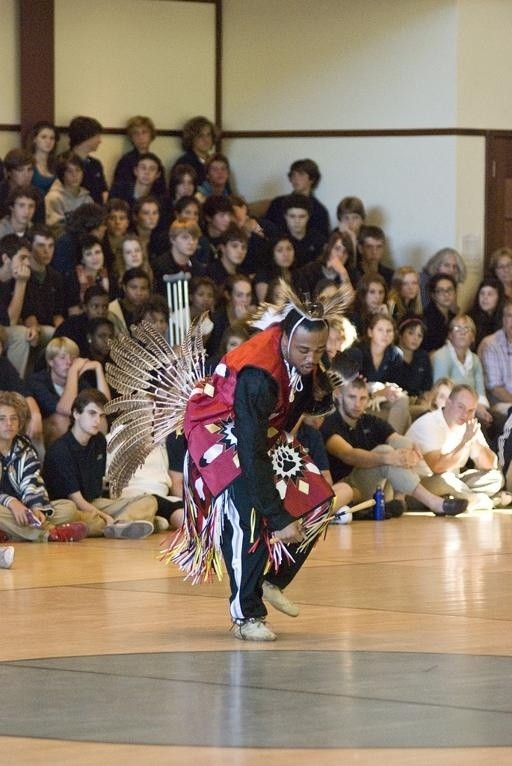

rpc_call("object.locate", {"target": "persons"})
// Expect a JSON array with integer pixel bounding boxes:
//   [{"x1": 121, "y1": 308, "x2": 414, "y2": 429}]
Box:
[
  {"x1": 37, "y1": 156, "x2": 94, "y2": 221},
  {"x1": 166, "y1": 165, "x2": 205, "y2": 204},
  {"x1": 435, "y1": 318, "x2": 489, "y2": 430},
  {"x1": 109, "y1": 270, "x2": 151, "y2": 333},
  {"x1": 465, "y1": 280, "x2": 500, "y2": 333},
  {"x1": 2, "y1": 193, "x2": 32, "y2": 235},
  {"x1": 56, "y1": 115, "x2": 110, "y2": 206},
  {"x1": 72, "y1": 229, "x2": 111, "y2": 304},
  {"x1": 286, "y1": 408, "x2": 348, "y2": 544},
  {"x1": 335, "y1": 197, "x2": 374, "y2": 263},
  {"x1": 114, "y1": 116, "x2": 163, "y2": 192},
  {"x1": 165, "y1": 283, "x2": 215, "y2": 349},
  {"x1": 351, "y1": 322, "x2": 402, "y2": 394},
  {"x1": 43, "y1": 391, "x2": 160, "y2": 538},
  {"x1": 0, "y1": 391, "x2": 85, "y2": 546},
  {"x1": 492, "y1": 399, "x2": 512, "y2": 509},
  {"x1": 357, "y1": 318, "x2": 402, "y2": 387},
  {"x1": 219, "y1": 279, "x2": 254, "y2": 330},
  {"x1": 210, "y1": 203, "x2": 239, "y2": 246},
  {"x1": 24, "y1": 225, "x2": 67, "y2": 317},
  {"x1": 276, "y1": 199, "x2": 320, "y2": 266},
  {"x1": 126, "y1": 298, "x2": 170, "y2": 379},
  {"x1": 4, "y1": 150, "x2": 38, "y2": 205},
  {"x1": 356, "y1": 223, "x2": 397, "y2": 282},
  {"x1": 108, "y1": 155, "x2": 167, "y2": 216},
  {"x1": 135, "y1": 199, "x2": 159, "y2": 247},
  {"x1": 416, "y1": 250, "x2": 464, "y2": 301},
  {"x1": 173, "y1": 117, "x2": 227, "y2": 182},
  {"x1": 20, "y1": 121, "x2": 56, "y2": 194},
  {"x1": 152, "y1": 228, "x2": 198, "y2": 286},
  {"x1": 77, "y1": 321, "x2": 115, "y2": 382},
  {"x1": 0, "y1": 237, "x2": 56, "y2": 380},
  {"x1": 109, "y1": 237, "x2": 149, "y2": 282},
  {"x1": 481, "y1": 247, "x2": 512, "y2": 295},
  {"x1": 203, "y1": 154, "x2": 238, "y2": 195},
  {"x1": 166, "y1": 384, "x2": 200, "y2": 536},
  {"x1": 382, "y1": 322, "x2": 430, "y2": 395},
  {"x1": 260, "y1": 276, "x2": 293, "y2": 320},
  {"x1": 382, "y1": 263, "x2": 422, "y2": 322},
  {"x1": 45, "y1": 205, "x2": 107, "y2": 285},
  {"x1": 94, "y1": 200, "x2": 131, "y2": 239},
  {"x1": 419, "y1": 278, "x2": 456, "y2": 335},
  {"x1": 202, "y1": 233, "x2": 253, "y2": 281},
  {"x1": 0, "y1": 329, "x2": 46, "y2": 471},
  {"x1": 170, "y1": 203, "x2": 205, "y2": 245},
  {"x1": 55, "y1": 291, "x2": 118, "y2": 341},
  {"x1": 479, "y1": 305, "x2": 512, "y2": 434},
  {"x1": 272, "y1": 159, "x2": 331, "y2": 245},
  {"x1": 159, "y1": 303, "x2": 336, "y2": 642},
  {"x1": 252, "y1": 238, "x2": 308, "y2": 300},
  {"x1": 243, "y1": 196, "x2": 275, "y2": 246},
  {"x1": 331, "y1": 382, "x2": 469, "y2": 517},
  {"x1": 341, "y1": 277, "x2": 388, "y2": 332},
  {"x1": 392, "y1": 385, "x2": 503, "y2": 506},
  {"x1": 32, "y1": 338, "x2": 109, "y2": 449},
  {"x1": 321, "y1": 232, "x2": 349, "y2": 289},
  {"x1": 210, "y1": 332, "x2": 242, "y2": 371}
]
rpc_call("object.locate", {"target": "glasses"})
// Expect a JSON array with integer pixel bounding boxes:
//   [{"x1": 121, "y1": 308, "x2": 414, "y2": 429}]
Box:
[
  {"x1": 431, "y1": 285, "x2": 457, "y2": 294},
  {"x1": 449, "y1": 325, "x2": 473, "y2": 336}
]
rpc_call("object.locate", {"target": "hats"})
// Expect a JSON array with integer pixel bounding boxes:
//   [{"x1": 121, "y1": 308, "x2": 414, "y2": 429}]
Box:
[
  {"x1": 69, "y1": 115, "x2": 103, "y2": 146},
  {"x1": 281, "y1": 193, "x2": 312, "y2": 216}
]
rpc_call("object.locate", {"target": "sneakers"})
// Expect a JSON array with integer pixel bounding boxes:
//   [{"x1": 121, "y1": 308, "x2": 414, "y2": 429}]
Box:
[
  {"x1": 103, "y1": 519, "x2": 155, "y2": 541},
  {"x1": 329, "y1": 506, "x2": 353, "y2": 524},
  {"x1": 153, "y1": 515, "x2": 169, "y2": 532},
  {"x1": 260, "y1": 578, "x2": 300, "y2": 618},
  {"x1": 47, "y1": 519, "x2": 89, "y2": 542},
  {"x1": 0, "y1": 546, "x2": 15, "y2": 567},
  {"x1": 230, "y1": 619, "x2": 279, "y2": 642}
]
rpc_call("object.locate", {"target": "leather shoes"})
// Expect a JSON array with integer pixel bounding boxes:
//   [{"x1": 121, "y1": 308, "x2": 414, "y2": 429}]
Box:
[
  {"x1": 432, "y1": 496, "x2": 469, "y2": 518},
  {"x1": 380, "y1": 497, "x2": 405, "y2": 520}
]
